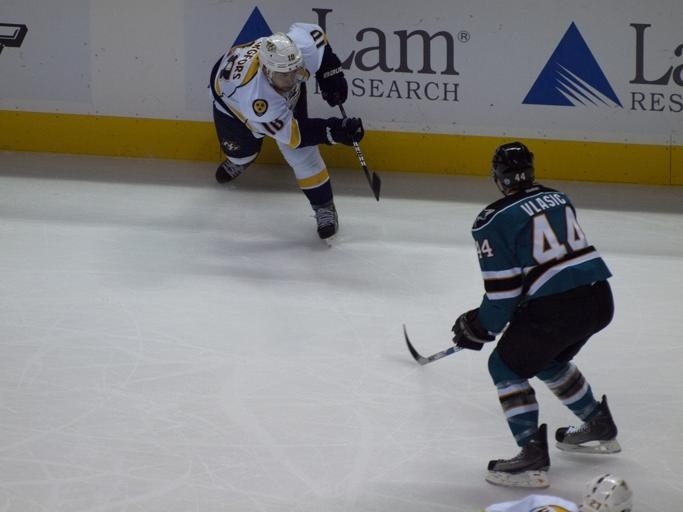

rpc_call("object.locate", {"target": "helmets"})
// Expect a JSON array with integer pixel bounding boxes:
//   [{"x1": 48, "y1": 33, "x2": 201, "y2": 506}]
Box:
[
  {"x1": 491, "y1": 141, "x2": 535, "y2": 190},
  {"x1": 579, "y1": 471, "x2": 632, "y2": 511},
  {"x1": 260, "y1": 32, "x2": 304, "y2": 84}
]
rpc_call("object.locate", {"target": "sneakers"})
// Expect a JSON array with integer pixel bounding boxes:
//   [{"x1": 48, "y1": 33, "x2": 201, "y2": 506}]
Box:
[
  {"x1": 215, "y1": 157, "x2": 253, "y2": 183},
  {"x1": 555, "y1": 392, "x2": 616, "y2": 444},
  {"x1": 313, "y1": 207, "x2": 339, "y2": 238},
  {"x1": 487, "y1": 424, "x2": 552, "y2": 473}
]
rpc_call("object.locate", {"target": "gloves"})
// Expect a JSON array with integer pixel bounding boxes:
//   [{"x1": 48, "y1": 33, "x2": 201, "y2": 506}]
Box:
[
  {"x1": 321, "y1": 113, "x2": 364, "y2": 147},
  {"x1": 315, "y1": 55, "x2": 348, "y2": 107},
  {"x1": 452, "y1": 309, "x2": 494, "y2": 351}
]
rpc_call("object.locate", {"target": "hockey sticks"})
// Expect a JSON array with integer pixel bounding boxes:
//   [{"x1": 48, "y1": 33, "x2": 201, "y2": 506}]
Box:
[
  {"x1": 337, "y1": 103, "x2": 380, "y2": 201},
  {"x1": 401, "y1": 322, "x2": 464, "y2": 366}
]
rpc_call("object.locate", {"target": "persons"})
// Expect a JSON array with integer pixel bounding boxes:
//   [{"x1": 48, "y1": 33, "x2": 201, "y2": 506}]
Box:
[
  {"x1": 453, "y1": 142, "x2": 618, "y2": 475},
  {"x1": 208, "y1": 21, "x2": 366, "y2": 239}
]
